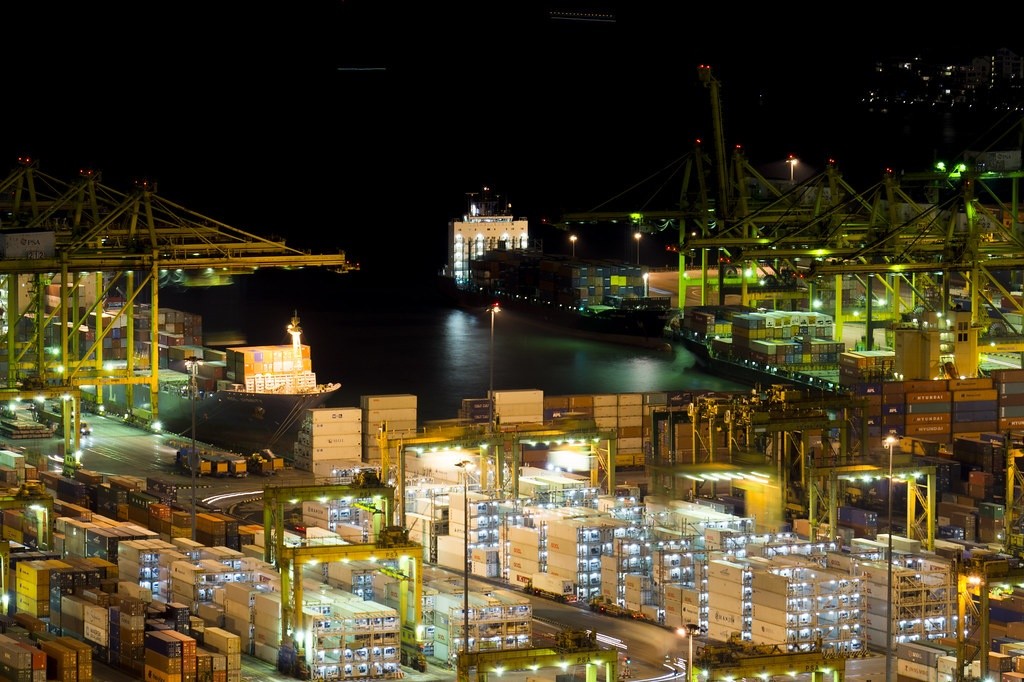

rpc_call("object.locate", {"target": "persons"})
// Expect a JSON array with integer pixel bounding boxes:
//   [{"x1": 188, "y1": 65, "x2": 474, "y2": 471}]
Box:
[{"x1": 674, "y1": 668, "x2": 678, "y2": 675}]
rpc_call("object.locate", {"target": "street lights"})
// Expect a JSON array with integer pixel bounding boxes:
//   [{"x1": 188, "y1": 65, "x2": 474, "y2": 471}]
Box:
[{"x1": 485, "y1": 303, "x2": 500, "y2": 434}]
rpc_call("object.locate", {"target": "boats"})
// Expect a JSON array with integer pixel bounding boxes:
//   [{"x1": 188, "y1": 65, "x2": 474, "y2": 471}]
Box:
[
  {"x1": 79, "y1": 297, "x2": 342, "y2": 467},
  {"x1": 437, "y1": 185, "x2": 680, "y2": 350},
  {"x1": 678, "y1": 301, "x2": 1024, "y2": 399}
]
